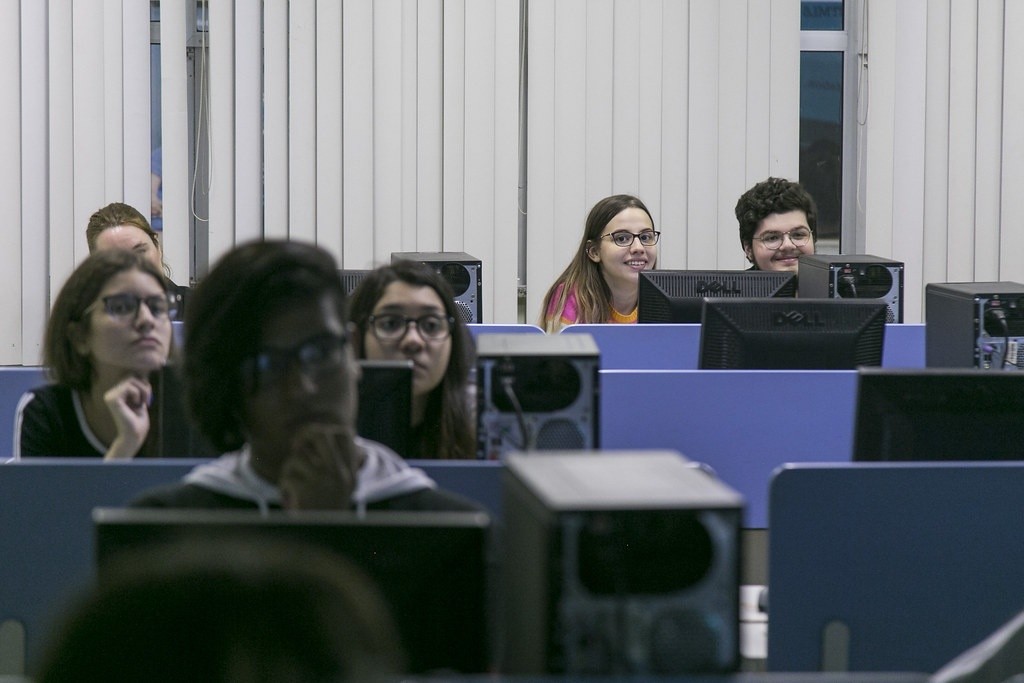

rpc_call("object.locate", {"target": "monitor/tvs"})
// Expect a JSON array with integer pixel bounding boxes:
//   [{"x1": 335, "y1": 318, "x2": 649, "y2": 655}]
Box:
[
  {"x1": 697, "y1": 296, "x2": 886, "y2": 370},
  {"x1": 852, "y1": 366, "x2": 1024, "y2": 462},
  {"x1": 155, "y1": 361, "x2": 414, "y2": 459},
  {"x1": 636, "y1": 270, "x2": 797, "y2": 323},
  {"x1": 91, "y1": 506, "x2": 491, "y2": 680}
]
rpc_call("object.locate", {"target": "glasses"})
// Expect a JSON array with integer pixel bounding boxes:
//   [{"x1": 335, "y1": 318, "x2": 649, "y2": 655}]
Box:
[
  {"x1": 598, "y1": 230, "x2": 659, "y2": 246},
  {"x1": 80, "y1": 292, "x2": 174, "y2": 321},
  {"x1": 229, "y1": 331, "x2": 351, "y2": 391},
  {"x1": 752, "y1": 225, "x2": 813, "y2": 249},
  {"x1": 365, "y1": 311, "x2": 456, "y2": 344}
]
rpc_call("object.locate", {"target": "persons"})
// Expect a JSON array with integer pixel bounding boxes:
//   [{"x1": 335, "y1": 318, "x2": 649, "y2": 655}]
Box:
[
  {"x1": 82, "y1": 201, "x2": 199, "y2": 322},
  {"x1": 21, "y1": 243, "x2": 194, "y2": 462},
  {"x1": 119, "y1": 237, "x2": 479, "y2": 518},
  {"x1": 536, "y1": 193, "x2": 663, "y2": 336},
  {"x1": 342, "y1": 260, "x2": 480, "y2": 462},
  {"x1": 735, "y1": 176, "x2": 821, "y2": 276}
]
